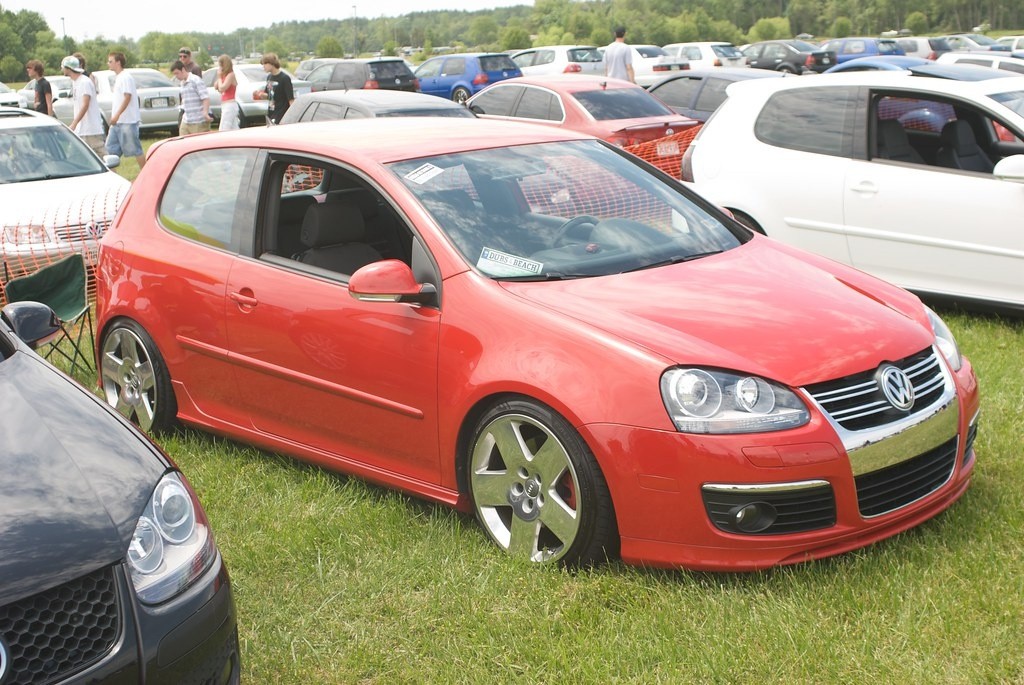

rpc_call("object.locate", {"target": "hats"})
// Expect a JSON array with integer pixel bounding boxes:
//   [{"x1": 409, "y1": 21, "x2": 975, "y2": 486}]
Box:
[{"x1": 61, "y1": 56, "x2": 85, "y2": 73}]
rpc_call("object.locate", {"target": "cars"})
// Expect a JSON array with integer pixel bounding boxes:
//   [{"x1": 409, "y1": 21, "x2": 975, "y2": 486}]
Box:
[
  {"x1": 1, "y1": 301, "x2": 241, "y2": 684},
  {"x1": 680, "y1": 66, "x2": 1023, "y2": 310},
  {"x1": 0, "y1": 102, "x2": 132, "y2": 288},
  {"x1": 91, "y1": 114, "x2": 979, "y2": 578},
  {"x1": 0, "y1": 33, "x2": 1023, "y2": 147}
]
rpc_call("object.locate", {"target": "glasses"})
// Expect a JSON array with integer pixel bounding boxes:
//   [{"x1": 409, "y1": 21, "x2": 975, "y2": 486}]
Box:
[{"x1": 179, "y1": 55, "x2": 187, "y2": 58}]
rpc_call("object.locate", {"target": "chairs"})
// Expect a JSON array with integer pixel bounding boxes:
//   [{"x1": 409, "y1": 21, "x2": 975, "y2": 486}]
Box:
[
  {"x1": 878, "y1": 116, "x2": 926, "y2": 163},
  {"x1": 294, "y1": 197, "x2": 384, "y2": 277},
  {"x1": 932, "y1": 118, "x2": 995, "y2": 172},
  {"x1": 423, "y1": 190, "x2": 478, "y2": 219},
  {"x1": 3, "y1": 246, "x2": 98, "y2": 376}
]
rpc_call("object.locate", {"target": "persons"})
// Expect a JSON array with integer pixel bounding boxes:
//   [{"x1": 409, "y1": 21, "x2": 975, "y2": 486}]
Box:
[
  {"x1": 259, "y1": 52, "x2": 294, "y2": 125},
  {"x1": 601, "y1": 25, "x2": 634, "y2": 84},
  {"x1": 171, "y1": 46, "x2": 214, "y2": 136},
  {"x1": 102, "y1": 51, "x2": 145, "y2": 170},
  {"x1": 213, "y1": 53, "x2": 241, "y2": 131},
  {"x1": 60, "y1": 53, "x2": 106, "y2": 164},
  {"x1": 26, "y1": 59, "x2": 57, "y2": 119}
]
